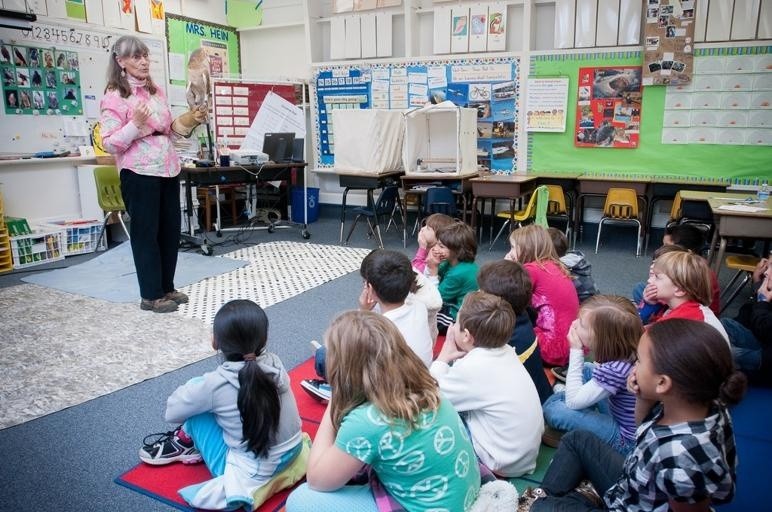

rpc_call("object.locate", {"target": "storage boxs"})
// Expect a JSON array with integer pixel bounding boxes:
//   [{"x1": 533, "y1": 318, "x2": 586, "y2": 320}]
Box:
[
  {"x1": 40, "y1": 215, "x2": 106, "y2": 257},
  {"x1": 8, "y1": 227, "x2": 65, "y2": 269}
]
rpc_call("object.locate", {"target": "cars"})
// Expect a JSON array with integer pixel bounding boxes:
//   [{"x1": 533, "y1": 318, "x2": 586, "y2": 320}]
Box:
[{"x1": 492, "y1": 145, "x2": 510, "y2": 154}]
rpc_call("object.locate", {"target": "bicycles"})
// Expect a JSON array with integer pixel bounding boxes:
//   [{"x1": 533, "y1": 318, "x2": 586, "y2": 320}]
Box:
[{"x1": 471, "y1": 86, "x2": 489, "y2": 99}]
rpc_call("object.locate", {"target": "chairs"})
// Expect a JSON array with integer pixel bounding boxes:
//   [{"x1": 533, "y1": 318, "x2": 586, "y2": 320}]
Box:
[
  {"x1": 343, "y1": 185, "x2": 397, "y2": 249},
  {"x1": 715, "y1": 255, "x2": 772, "y2": 317},
  {"x1": 90, "y1": 166, "x2": 132, "y2": 253},
  {"x1": 595, "y1": 188, "x2": 647, "y2": 257},
  {"x1": 402, "y1": 173, "x2": 576, "y2": 255}
]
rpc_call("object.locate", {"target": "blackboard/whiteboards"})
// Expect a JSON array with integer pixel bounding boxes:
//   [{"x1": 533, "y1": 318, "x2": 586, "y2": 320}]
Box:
[
  {"x1": 1, "y1": 13, "x2": 173, "y2": 166},
  {"x1": 207, "y1": 75, "x2": 307, "y2": 158},
  {"x1": 311, "y1": 44, "x2": 772, "y2": 188}
]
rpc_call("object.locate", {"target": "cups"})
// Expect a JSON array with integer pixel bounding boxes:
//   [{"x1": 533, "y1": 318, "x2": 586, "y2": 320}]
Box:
[{"x1": 219, "y1": 154, "x2": 230, "y2": 167}]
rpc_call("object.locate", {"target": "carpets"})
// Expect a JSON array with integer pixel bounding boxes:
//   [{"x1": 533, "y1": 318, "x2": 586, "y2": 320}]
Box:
[
  {"x1": 0, "y1": 281, "x2": 222, "y2": 433},
  {"x1": 19, "y1": 234, "x2": 252, "y2": 304},
  {"x1": 114, "y1": 329, "x2": 460, "y2": 512},
  {"x1": 174, "y1": 237, "x2": 373, "y2": 328}
]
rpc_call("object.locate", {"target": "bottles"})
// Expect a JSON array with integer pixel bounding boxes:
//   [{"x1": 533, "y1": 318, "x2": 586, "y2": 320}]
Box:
[
  {"x1": 200, "y1": 132, "x2": 207, "y2": 146},
  {"x1": 756, "y1": 178, "x2": 769, "y2": 207}
]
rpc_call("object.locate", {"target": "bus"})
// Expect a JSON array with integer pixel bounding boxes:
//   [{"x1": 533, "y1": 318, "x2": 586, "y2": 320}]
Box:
[{"x1": 493, "y1": 85, "x2": 514, "y2": 98}]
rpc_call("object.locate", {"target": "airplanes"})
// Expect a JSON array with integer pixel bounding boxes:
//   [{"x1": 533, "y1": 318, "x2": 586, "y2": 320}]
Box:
[{"x1": 448, "y1": 88, "x2": 463, "y2": 98}]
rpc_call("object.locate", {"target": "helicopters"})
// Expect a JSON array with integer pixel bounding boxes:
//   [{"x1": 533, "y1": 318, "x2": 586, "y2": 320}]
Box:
[{"x1": 494, "y1": 108, "x2": 509, "y2": 117}]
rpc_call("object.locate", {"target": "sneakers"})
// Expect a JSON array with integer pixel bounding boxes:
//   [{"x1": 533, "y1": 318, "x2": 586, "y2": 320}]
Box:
[
  {"x1": 299, "y1": 378, "x2": 332, "y2": 405},
  {"x1": 138, "y1": 425, "x2": 203, "y2": 466},
  {"x1": 140, "y1": 290, "x2": 188, "y2": 313},
  {"x1": 551, "y1": 366, "x2": 568, "y2": 382},
  {"x1": 517, "y1": 487, "x2": 549, "y2": 512}
]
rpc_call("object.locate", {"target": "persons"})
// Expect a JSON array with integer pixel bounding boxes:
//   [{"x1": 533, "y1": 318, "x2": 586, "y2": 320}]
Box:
[
  {"x1": 97, "y1": 35, "x2": 210, "y2": 313},
  {"x1": 139, "y1": 214, "x2": 771, "y2": 511}
]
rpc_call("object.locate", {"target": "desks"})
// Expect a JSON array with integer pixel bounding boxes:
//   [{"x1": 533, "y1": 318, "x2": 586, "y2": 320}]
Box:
[
  {"x1": 705, "y1": 194, "x2": 772, "y2": 279},
  {"x1": 646, "y1": 181, "x2": 731, "y2": 254},
  {"x1": 667, "y1": 191, "x2": 772, "y2": 247},
  {"x1": 0, "y1": 153, "x2": 122, "y2": 250},
  {"x1": 335, "y1": 168, "x2": 401, "y2": 250},
  {"x1": 577, "y1": 174, "x2": 650, "y2": 261}
]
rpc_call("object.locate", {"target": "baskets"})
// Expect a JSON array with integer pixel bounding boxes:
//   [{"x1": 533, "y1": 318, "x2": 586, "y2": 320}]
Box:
[{"x1": 9, "y1": 217, "x2": 109, "y2": 270}]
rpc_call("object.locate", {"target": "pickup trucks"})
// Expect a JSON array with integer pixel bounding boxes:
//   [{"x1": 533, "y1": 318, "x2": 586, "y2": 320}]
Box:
[
  {"x1": 477, "y1": 147, "x2": 489, "y2": 157},
  {"x1": 478, "y1": 164, "x2": 490, "y2": 171}
]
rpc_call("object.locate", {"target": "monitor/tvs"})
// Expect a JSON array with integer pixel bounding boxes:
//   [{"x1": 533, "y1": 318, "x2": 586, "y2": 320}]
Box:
[{"x1": 262, "y1": 133, "x2": 293, "y2": 164}]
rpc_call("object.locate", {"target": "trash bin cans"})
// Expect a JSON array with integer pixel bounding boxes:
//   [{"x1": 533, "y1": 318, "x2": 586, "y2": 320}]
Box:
[{"x1": 291, "y1": 186, "x2": 320, "y2": 224}]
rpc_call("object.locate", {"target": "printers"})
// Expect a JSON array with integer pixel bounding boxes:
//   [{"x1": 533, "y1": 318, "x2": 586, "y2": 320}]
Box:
[{"x1": 229, "y1": 149, "x2": 270, "y2": 165}]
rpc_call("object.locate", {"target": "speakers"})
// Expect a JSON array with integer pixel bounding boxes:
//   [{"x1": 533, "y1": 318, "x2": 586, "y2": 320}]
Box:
[{"x1": 294, "y1": 139, "x2": 304, "y2": 162}]
